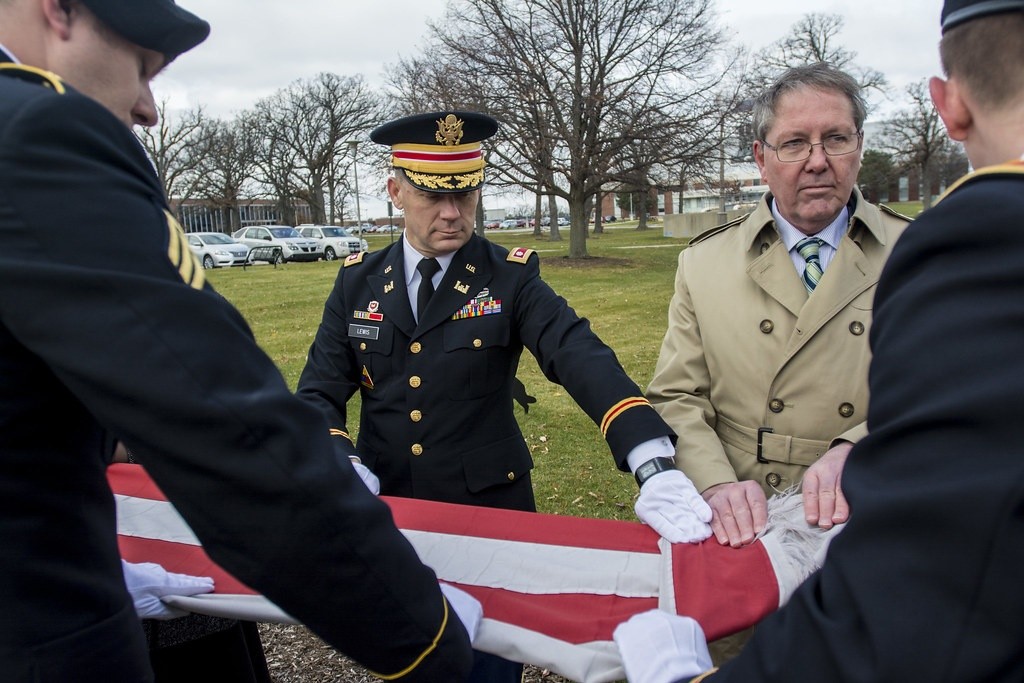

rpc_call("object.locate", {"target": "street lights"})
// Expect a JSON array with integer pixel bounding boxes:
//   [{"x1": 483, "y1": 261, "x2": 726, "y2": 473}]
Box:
[{"x1": 345, "y1": 140, "x2": 363, "y2": 253}]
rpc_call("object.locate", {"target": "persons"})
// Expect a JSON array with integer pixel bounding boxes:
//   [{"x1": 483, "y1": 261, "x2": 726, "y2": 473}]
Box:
[
  {"x1": 293, "y1": 111, "x2": 712, "y2": 683},
  {"x1": 0, "y1": 0, "x2": 486, "y2": 683},
  {"x1": 612, "y1": 0, "x2": 1024, "y2": 683},
  {"x1": 644, "y1": 61, "x2": 916, "y2": 666}
]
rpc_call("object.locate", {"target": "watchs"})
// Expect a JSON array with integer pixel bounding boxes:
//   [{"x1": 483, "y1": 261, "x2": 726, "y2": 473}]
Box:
[{"x1": 635, "y1": 457, "x2": 677, "y2": 490}]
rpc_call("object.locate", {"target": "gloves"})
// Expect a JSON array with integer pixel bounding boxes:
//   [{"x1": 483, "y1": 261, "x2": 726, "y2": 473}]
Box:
[
  {"x1": 439, "y1": 582, "x2": 483, "y2": 646},
  {"x1": 634, "y1": 469, "x2": 713, "y2": 544},
  {"x1": 352, "y1": 462, "x2": 380, "y2": 496},
  {"x1": 612, "y1": 609, "x2": 713, "y2": 682},
  {"x1": 121, "y1": 558, "x2": 214, "y2": 620}
]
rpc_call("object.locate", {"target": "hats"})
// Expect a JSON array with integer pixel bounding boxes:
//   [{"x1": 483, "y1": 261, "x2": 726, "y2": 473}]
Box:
[
  {"x1": 85, "y1": 0, "x2": 210, "y2": 66},
  {"x1": 940, "y1": 0, "x2": 1024, "y2": 36},
  {"x1": 370, "y1": 112, "x2": 498, "y2": 193}
]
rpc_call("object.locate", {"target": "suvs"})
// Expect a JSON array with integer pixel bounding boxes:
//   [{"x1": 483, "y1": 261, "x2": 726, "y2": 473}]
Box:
[
  {"x1": 291, "y1": 223, "x2": 369, "y2": 261},
  {"x1": 230, "y1": 225, "x2": 322, "y2": 266}
]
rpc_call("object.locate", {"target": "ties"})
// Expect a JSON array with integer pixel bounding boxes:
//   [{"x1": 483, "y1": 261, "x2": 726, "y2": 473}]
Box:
[
  {"x1": 417, "y1": 257, "x2": 441, "y2": 325},
  {"x1": 795, "y1": 237, "x2": 825, "y2": 297}
]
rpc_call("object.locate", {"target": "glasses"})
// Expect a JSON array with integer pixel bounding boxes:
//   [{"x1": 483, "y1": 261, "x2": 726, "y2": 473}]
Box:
[{"x1": 762, "y1": 132, "x2": 861, "y2": 162}]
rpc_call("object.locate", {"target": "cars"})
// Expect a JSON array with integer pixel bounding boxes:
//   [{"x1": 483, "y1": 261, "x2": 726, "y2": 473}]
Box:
[
  {"x1": 474, "y1": 214, "x2": 618, "y2": 231},
  {"x1": 346, "y1": 221, "x2": 378, "y2": 234},
  {"x1": 185, "y1": 232, "x2": 256, "y2": 270},
  {"x1": 376, "y1": 224, "x2": 399, "y2": 235}
]
